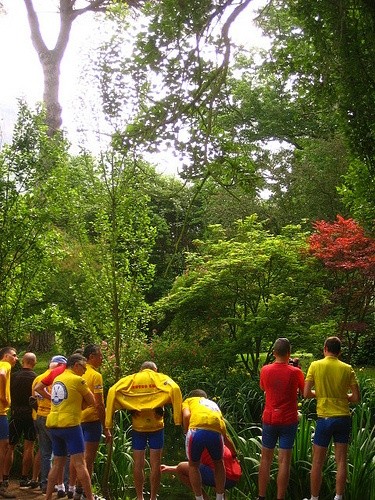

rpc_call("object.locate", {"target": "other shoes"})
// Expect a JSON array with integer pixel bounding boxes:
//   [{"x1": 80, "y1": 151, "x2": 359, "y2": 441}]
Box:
[
  {"x1": 19, "y1": 478, "x2": 30, "y2": 489},
  {"x1": 57, "y1": 490, "x2": 65, "y2": 497},
  {"x1": 4, "y1": 475, "x2": 9, "y2": 481},
  {"x1": 30, "y1": 481, "x2": 41, "y2": 490},
  {"x1": 68, "y1": 491, "x2": 73, "y2": 498}
]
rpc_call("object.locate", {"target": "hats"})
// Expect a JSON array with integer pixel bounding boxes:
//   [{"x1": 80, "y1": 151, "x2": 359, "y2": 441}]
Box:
[{"x1": 51, "y1": 355, "x2": 67, "y2": 363}]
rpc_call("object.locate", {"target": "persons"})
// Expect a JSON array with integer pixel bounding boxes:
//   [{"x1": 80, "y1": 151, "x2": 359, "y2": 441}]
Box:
[
  {"x1": 105, "y1": 362, "x2": 182, "y2": 500},
  {"x1": 182, "y1": 389, "x2": 237, "y2": 500},
  {"x1": 31, "y1": 344, "x2": 112, "y2": 500},
  {"x1": 3, "y1": 353, "x2": 40, "y2": 489},
  {"x1": 160, "y1": 445, "x2": 242, "y2": 500},
  {"x1": 46, "y1": 354, "x2": 95, "y2": 500},
  {"x1": 0, "y1": 347, "x2": 19, "y2": 500},
  {"x1": 258, "y1": 338, "x2": 306, "y2": 500},
  {"x1": 304, "y1": 336, "x2": 361, "y2": 500}
]
